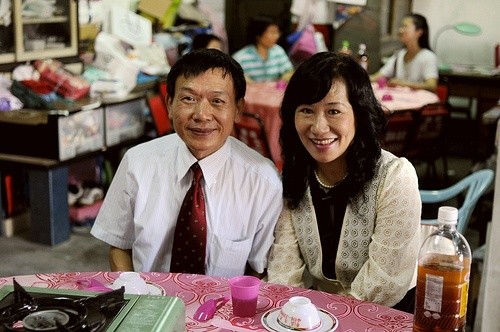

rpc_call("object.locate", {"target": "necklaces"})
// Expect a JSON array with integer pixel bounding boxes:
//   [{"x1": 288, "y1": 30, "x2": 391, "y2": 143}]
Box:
[{"x1": 314, "y1": 171, "x2": 348, "y2": 188}]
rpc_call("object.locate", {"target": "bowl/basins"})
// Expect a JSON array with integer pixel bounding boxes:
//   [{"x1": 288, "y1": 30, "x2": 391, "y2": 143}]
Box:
[
  {"x1": 278, "y1": 296, "x2": 320, "y2": 329},
  {"x1": 112, "y1": 272, "x2": 150, "y2": 295}
]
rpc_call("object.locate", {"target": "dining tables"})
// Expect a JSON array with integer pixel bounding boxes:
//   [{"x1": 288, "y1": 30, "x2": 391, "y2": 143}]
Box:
[
  {"x1": 0, "y1": 273, "x2": 415, "y2": 332},
  {"x1": 241, "y1": 81, "x2": 440, "y2": 175}
]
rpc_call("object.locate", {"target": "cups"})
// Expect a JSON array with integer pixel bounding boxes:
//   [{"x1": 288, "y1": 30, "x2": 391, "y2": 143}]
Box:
[{"x1": 229, "y1": 275, "x2": 261, "y2": 317}]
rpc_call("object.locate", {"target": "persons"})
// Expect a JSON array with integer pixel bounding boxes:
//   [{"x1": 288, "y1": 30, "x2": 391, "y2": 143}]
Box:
[
  {"x1": 191, "y1": 34, "x2": 223, "y2": 50},
  {"x1": 230, "y1": 17, "x2": 295, "y2": 82},
  {"x1": 90, "y1": 48, "x2": 282, "y2": 278},
  {"x1": 368, "y1": 13, "x2": 439, "y2": 91},
  {"x1": 267, "y1": 51, "x2": 422, "y2": 315}
]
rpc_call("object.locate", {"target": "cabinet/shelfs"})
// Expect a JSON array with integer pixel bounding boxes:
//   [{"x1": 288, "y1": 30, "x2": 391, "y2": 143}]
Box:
[{"x1": 0, "y1": 0, "x2": 78, "y2": 65}]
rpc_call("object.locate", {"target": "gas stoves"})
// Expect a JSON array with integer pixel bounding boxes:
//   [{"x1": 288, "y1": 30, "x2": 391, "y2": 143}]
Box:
[{"x1": 0, "y1": 280, "x2": 186, "y2": 332}]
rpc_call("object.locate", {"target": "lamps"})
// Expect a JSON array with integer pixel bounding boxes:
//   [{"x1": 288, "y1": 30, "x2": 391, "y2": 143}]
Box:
[{"x1": 431, "y1": 22, "x2": 480, "y2": 71}]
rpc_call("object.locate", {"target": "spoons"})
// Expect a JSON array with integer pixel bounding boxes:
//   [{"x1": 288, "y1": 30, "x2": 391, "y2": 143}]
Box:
[{"x1": 193, "y1": 295, "x2": 230, "y2": 322}]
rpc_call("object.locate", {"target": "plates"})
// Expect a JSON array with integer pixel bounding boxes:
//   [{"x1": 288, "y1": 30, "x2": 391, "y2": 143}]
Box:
[
  {"x1": 106, "y1": 282, "x2": 166, "y2": 296},
  {"x1": 261, "y1": 307, "x2": 339, "y2": 332}
]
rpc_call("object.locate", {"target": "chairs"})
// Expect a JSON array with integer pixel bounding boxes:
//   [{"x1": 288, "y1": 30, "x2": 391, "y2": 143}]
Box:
[
  {"x1": 234, "y1": 112, "x2": 272, "y2": 163},
  {"x1": 419, "y1": 169, "x2": 494, "y2": 244}
]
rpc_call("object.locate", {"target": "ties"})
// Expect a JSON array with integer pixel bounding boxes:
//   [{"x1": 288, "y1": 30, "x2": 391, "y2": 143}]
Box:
[{"x1": 169, "y1": 162, "x2": 209, "y2": 274}]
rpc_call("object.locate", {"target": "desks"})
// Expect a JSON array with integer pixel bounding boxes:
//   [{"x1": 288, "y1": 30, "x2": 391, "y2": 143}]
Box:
[{"x1": 445, "y1": 62, "x2": 500, "y2": 161}]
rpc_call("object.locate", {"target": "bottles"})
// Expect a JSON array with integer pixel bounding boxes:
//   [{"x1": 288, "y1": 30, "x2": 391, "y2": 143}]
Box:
[
  {"x1": 355, "y1": 43, "x2": 369, "y2": 71},
  {"x1": 412, "y1": 206, "x2": 472, "y2": 332},
  {"x1": 336, "y1": 40, "x2": 354, "y2": 57}
]
